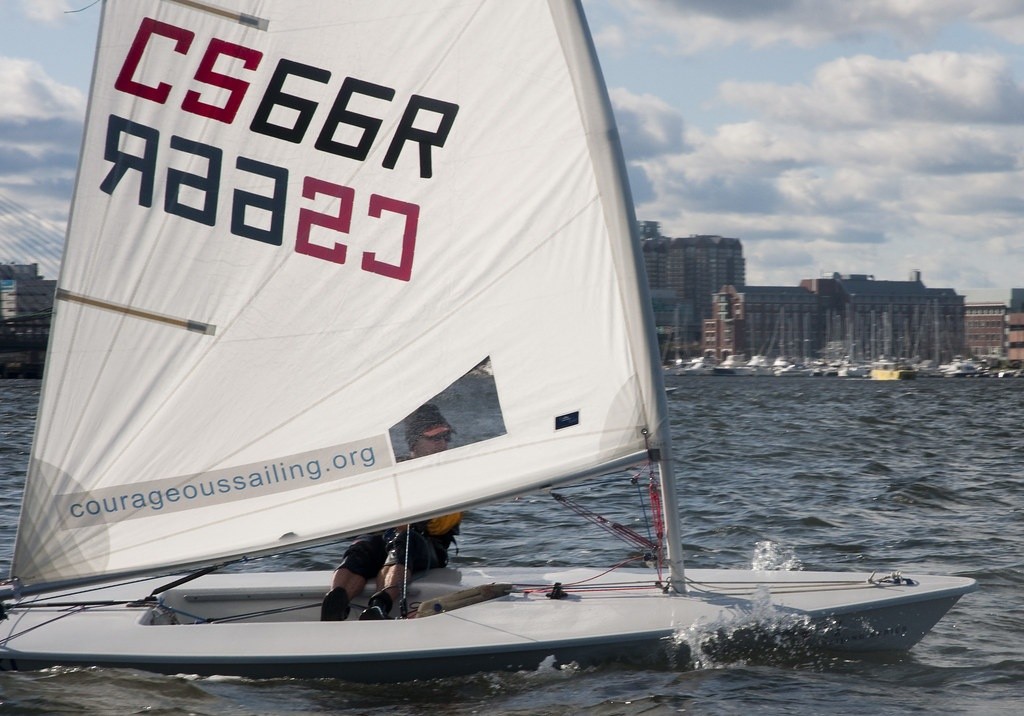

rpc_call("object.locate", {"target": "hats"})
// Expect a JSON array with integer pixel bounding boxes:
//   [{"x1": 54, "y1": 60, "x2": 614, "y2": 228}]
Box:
[{"x1": 405, "y1": 403, "x2": 457, "y2": 451}]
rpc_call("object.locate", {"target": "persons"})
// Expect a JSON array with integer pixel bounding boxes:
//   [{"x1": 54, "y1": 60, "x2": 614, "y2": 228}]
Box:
[{"x1": 321, "y1": 404, "x2": 462, "y2": 621}]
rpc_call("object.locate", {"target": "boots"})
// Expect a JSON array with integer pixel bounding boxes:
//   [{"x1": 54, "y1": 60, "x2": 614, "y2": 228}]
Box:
[
  {"x1": 320, "y1": 586, "x2": 351, "y2": 621},
  {"x1": 359, "y1": 591, "x2": 392, "y2": 620}
]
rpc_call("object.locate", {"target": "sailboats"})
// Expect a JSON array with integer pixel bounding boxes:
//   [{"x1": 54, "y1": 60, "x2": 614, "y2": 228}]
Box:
[
  {"x1": 0, "y1": 0, "x2": 976, "y2": 688},
  {"x1": 664, "y1": 302, "x2": 1021, "y2": 381}
]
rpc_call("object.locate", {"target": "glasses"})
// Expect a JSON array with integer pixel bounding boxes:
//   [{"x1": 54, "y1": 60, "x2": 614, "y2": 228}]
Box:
[{"x1": 418, "y1": 433, "x2": 452, "y2": 442}]
